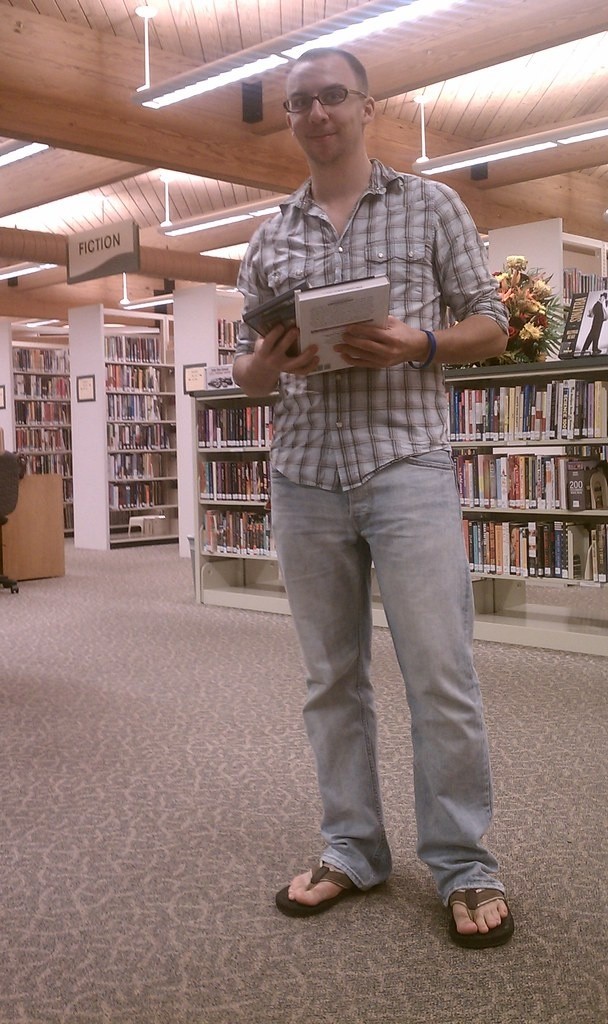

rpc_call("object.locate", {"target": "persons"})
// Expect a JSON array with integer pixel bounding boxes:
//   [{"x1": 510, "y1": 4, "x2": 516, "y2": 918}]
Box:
[
  {"x1": 230, "y1": 44, "x2": 515, "y2": 951},
  {"x1": 578, "y1": 291, "x2": 608, "y2": 358}
]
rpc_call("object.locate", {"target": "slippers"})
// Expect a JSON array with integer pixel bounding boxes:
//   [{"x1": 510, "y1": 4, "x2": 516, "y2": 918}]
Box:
[
  {"x1": 276, "y1": 864, "x2": 381, "y2": 918},
  {"x1": 448, "y1": 886, "x2": 516, "y2": 947}
]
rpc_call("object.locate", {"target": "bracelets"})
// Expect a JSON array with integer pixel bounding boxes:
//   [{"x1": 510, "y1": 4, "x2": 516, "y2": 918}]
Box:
[{"x1": 406, "y1": 329, "x2": 438, "y2": 371}]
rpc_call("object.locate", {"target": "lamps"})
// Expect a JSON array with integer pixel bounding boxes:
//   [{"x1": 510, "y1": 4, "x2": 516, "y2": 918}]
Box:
[
  {"x1": 0, "y1": 135, "x2": 52, "y2": 172},
  {"x1": 120, "y1": 271, "x2": 175, "y2": 309},
  {"x1": 135, "y1": 1, "x2": 466, "y2": 111},
  {"x1": 17, "y1": 318, "x2": 61, "y2": 330},
  {"x1": 413, "y1": 95, "x2": 607, "y2": 178},
  {"x1": 158, "y1": 168, "x2": 295, "y2": 239},
  {"x1": 0, "y1": 256, "x2": 58, "y2": 284}
]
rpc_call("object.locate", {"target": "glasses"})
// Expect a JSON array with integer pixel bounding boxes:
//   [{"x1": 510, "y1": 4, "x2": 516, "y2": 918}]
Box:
[{"x1": 283, "y1": 85, "x2": 366, "y2": 113}]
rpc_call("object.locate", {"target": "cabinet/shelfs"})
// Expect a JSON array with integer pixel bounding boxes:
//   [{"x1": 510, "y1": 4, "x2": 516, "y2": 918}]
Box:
[
  {"x1": 178, "y1": 345, "x2": 608, "y2": 655},
  {"x1": 485, "y1": 217, "x2": 608, "y2": 358},
  {"x1": 2, "y1": 315, "x2": 76, "y2": 534},
  {"x1": 65, "y1": 304, "x2": 179, "y2": 555},
  {"x1": 170, "y1": 277, "x2": 268, "y2": 559}
]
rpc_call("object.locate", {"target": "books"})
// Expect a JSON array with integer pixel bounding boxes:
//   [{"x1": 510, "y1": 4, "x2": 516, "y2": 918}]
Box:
[
  {"x1": 562, "y1": 261, "x2": 607, "y2": 320},
  {"x1": 8, "y1": 305, "x2": 271, "y2": 552},
  {"x1": 293, "y1": 272, "x2": 390, "y2": 377},
  {"x1": 243, "y1": 280, "x2": 320, "y2": 360},
  {"x1": 192, "y1": 373, "x2": 608, "y2": 608}
]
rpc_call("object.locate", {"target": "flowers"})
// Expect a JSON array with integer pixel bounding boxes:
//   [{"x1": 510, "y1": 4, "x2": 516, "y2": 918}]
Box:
[{"x1": 480, "y1": 258, "x2": 566, "y2": 365}]
